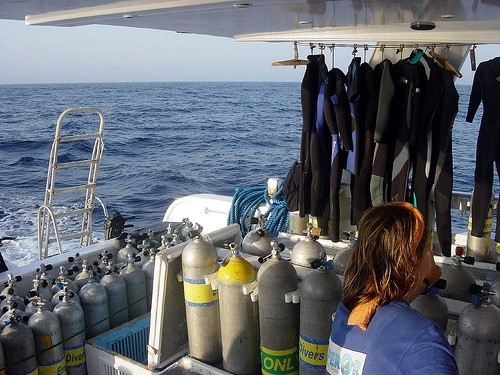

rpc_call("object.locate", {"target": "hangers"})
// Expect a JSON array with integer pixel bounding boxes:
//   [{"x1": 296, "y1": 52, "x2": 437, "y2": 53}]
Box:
[{"x1": 270, "y1": 41, "x2": 500, "y2": 90}]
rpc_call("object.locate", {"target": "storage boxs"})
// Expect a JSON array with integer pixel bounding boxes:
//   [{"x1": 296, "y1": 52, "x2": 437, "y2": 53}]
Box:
[{"x1": 84, "y1": 223, "x2": 241, "y2": 375}]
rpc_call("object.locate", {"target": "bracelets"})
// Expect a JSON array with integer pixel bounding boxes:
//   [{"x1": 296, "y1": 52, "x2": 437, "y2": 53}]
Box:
[{"x1": 423, "y1": 277, "x2": 430, "y2": 287}]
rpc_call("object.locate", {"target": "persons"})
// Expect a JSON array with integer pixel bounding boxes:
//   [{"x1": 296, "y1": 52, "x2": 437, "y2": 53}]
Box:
[{"x1": 325, "y1": 201, "x2": 462, "y2": 375}]
